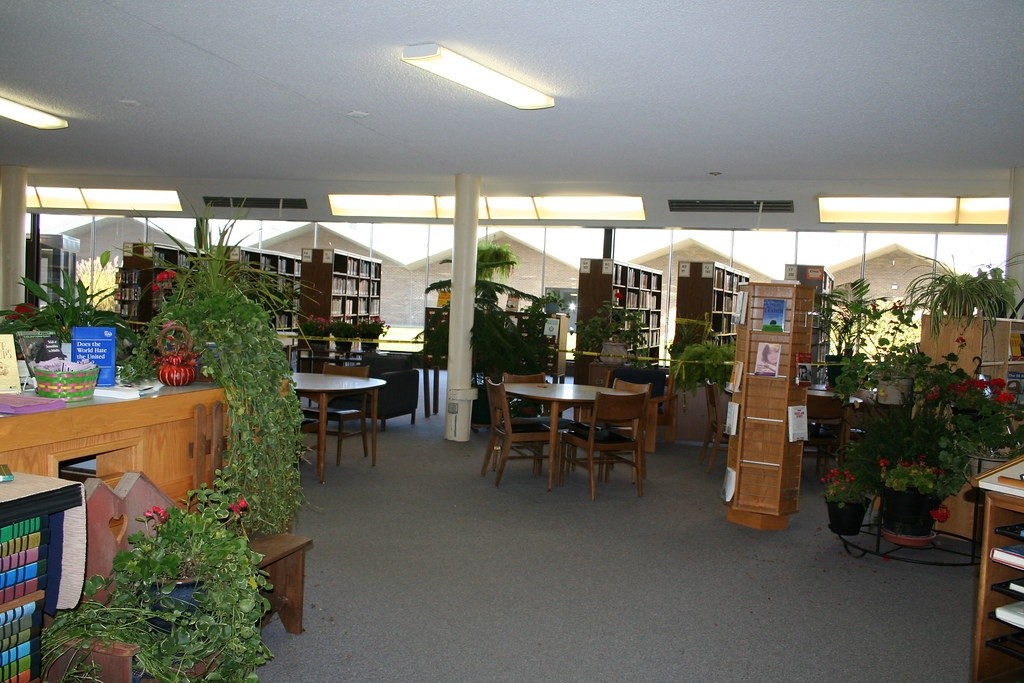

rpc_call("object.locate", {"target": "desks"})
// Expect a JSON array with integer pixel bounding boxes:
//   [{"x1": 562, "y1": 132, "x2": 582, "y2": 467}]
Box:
[
  {"x1": 297, "y1": 347, "x2": 439, "y2": 419},
  {"x1": 808, "y1": 390, "x2": 864, "y2": 408},
  {"x1": 288, "y1": 373, "x2": 387, "y2": 485},
  {"x1": 0, "y1": 473, "x2": 87, "y2": 683},
  {"x1": 504, "y1": 383, "x2": 639, "y2": 492}
]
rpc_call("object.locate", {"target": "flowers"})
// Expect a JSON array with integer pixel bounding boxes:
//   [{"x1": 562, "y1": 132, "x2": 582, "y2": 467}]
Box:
[
  {"x1": 296, "y1": 313, "x2": 331, "y2": 337},
  {"x1": 0, "y1": 185, "x2": 324, "y2": 683},
  {"x1": 81, "y1": 494, "x2": 276, "y2": 683},
  {"x1": 818, "y1": 333, "x2": 1024, "y2": 524},
  {"x1": 354, "y1": 315, "x2": 391, "y2": 337},
  {"x1": 569, "y1": 288, "x2": 651, "y2": 370}
]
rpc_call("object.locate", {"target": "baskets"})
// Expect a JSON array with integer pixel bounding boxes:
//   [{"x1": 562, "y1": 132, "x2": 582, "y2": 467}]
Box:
[{"x1": 33, "y1": 365, "x2": 100, "y2": 402}]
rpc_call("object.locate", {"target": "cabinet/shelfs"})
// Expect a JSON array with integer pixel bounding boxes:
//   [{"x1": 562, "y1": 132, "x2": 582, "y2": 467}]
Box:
[
  {"x1": 297, "y1": 247, "x2": 382, "y2": 357},
  {"x1": 674, "y1": 260, "x2": 751, "y2": 347},
  {"x1": 970, "y1": 453, "x2": 1024, "y2": 683},
  {"x1": 515, "y1": 312, "x2": 569, "y2": 384},
  {"x1": 211, "y1": 244, "x2": 302, "y2": 369},
  {"x1": 572, "y1": 258, "x2": 663, "y2": 385},
  {"x1": 911, "y1": 314, "x2": 1024, "y2": 543},
  {"x1": 784, "y1": 263, "x2": 836, "y2": 386},
  {"x1": 122, "y1": 241, "x2": 205, "y2": 354}
]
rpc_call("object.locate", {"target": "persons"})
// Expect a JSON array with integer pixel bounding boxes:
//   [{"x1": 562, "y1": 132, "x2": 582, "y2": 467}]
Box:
[
  {"x1": 756, "y1": 344, "x2": 779, "y2": 374},
  {"x1": 800, "y1": 366, "x2": 810, "y2": 381}
]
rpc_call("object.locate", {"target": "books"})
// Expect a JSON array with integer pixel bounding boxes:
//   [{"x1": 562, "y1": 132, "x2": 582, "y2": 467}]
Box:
[
  {"x1": 241, "y1": 250, "x2": 302, "y2": 329},
  {"x1": 113, "y1": 269, "x2": 142, "y2": 316},
  {"x1": 332, "y1": 254, "x2": 379, "y2": 318},
  {"x1": 816, "y1": 272, "x2": 833, "y2": 385},
  {"x1": 612, "y1": 264, "x2": 661, "y2": 350},
  {"x1": 755, "y1": 342, "x2": 781, "y2": 378},
  {"x1": 989, "y1": 542, "x2": 1024, "y2": 628},
  {"x1": 761, "y1": 298, "x2": 786, "y2": 333},
  {"x1": 1007, "y1": 331, "x2": 1024, "y2": 411},
  {"x1": 713, "y1": 268, "x2": 749, "y2": 345},
  {"x1": 788, "y1": 405, "x2": 808, "y2": 443},
  {"x1": 795, "y1": 351, "x2": 813, "y2": 387},
  {"x1": 724, "y1": 402, "x2": 741, "y2": 437},
  {"x1": 728, "y1": 359, "x2": 744, "y2": 393}
]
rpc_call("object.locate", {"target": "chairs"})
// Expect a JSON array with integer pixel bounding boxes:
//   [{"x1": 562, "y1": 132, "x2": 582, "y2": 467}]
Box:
[
  {"x1": 566, "y1": 378, "x2": 653, "y2": 485},
  {"x1": 480, "y1": 375, "x2": 562, "y2": 488},
  {"x1": 559, "y1": 391, "x2": 651, "y2": 502},
  {"x1": 803, "y1": 394, "x2": 849, "y2": 476},
  {"x1": 580, "y1": 368, "x2": 675, "y2": 453},
  {"x1": 697, "y1": 377, "x2": 730, "y2": 476},
  {"x1": 348, "y1": 354, "x2": 419, "y2": 432},
  {"x1": 491, "y1": 372, "x2": 545, "y2": 473},
  {"x1": 300, "y1": 362, "x2": 370, "y2": 467}
]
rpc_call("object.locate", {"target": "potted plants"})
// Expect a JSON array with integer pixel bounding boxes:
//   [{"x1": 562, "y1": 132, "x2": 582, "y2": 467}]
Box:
[
  {"x1": 506, "y1": 301, "x2": 556, "y2": 418},
  {"x1": 477, "y1": 235, "x2": 520, "y2": 280},
  {"x1": 668, "y1": 311, "x2": 714, "y2": 360},
  {"x1": 540, "y1": 290, "x2": 570, "y2": 314},
  {"x1": 816, "y1": 278, "x2": 891, "y2": 388},
  {"x1": 412, "y1": 237, "x2": 539, "y2": 424},
  {"x1": 977, "y1": 267, "x2": 1019, "y2": 319},
  {"x1": 830, "y1": 337, "x2": 933, "y2": 409},
  {"x1": 901, "y1": 252, "x2": 1024, "y2": 341},
  {"x1": 330, "y1": 320, "x2": 356, "y2": 352}
]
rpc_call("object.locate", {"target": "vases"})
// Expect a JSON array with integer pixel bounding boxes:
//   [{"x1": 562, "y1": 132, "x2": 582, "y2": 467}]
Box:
[
  {"x1": 360, "y1": 334, "x2": 379, "y2": 353},
  {"x1": 600, "y1": 341, "x2": 628, "y2": 366},
  {"x1": 884, "y1": 487, "x2": 949, "y2": 536},
  {"x1": 134, "y1": 577, "x2": 211, "y2": 642},
  {"x1": 950, "y1": 405, "x2": 996, "y2": 437},
  {"x1": 824, "y1": 496, "x2": 872, "y2": 536},
  {"x1": 308, "y1": 340, "x2": 329, "y2": 357},
  {"x1": 968, "y1": 454, "x2": 1010, "y2": 476}
]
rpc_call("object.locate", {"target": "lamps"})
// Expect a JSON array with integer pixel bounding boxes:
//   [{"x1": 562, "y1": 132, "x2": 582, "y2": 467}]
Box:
[
  {"x1": 400, "y1": 43, "x2": 556, "y2": 111},
  {"x1": 0, "y1": 97, "x2": 68, "y2": 130}
]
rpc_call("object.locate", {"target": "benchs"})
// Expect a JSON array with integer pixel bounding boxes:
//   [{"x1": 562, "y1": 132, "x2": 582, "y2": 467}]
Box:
[{"x1": 154, "y1": 531, "x2": 314, "y2": 634}]
